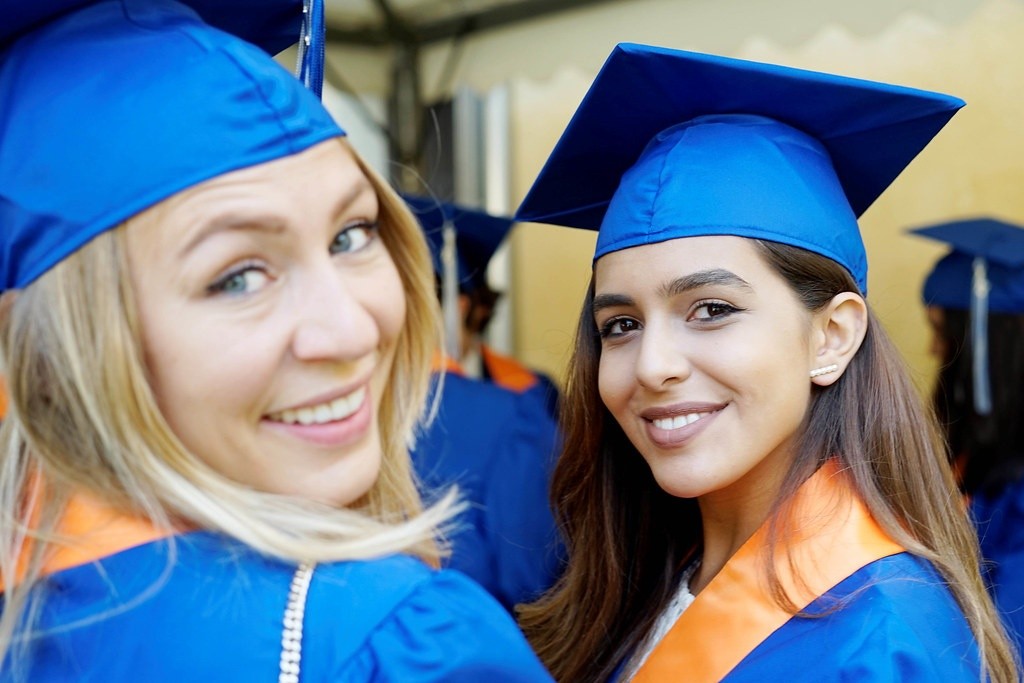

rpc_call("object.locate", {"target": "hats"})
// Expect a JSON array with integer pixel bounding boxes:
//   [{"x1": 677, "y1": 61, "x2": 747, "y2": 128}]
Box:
[
  {"x1": 0, "y1": 0, "x2": 346, "y2": 299},
  {"x1": 511, "y1": 42, "x2": 966, "y2": 299},
  {"x1": 907, "y1": 217, "x2": 1023, "y2": 414},
  {"x1": 398, "y1": 191, "x2": 515, "y2": 362}
]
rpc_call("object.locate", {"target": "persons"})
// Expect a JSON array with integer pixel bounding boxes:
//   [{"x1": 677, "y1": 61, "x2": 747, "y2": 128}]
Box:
[
  {"x1": 556, "y1": 41, "x2": 1020, "y2": 683},
  {"x1": 0, "y1": 0, "x2": 549, "y2": 682},
  {"x1": 462, "y1": 273, "x2": 560, "y2": 419},
  {"x1": 401, "y1": 196, "x2": 569, "y2": 618},
  {"x1": 910, "y1": 218, "x2": 1024, "y2": 683}
]
